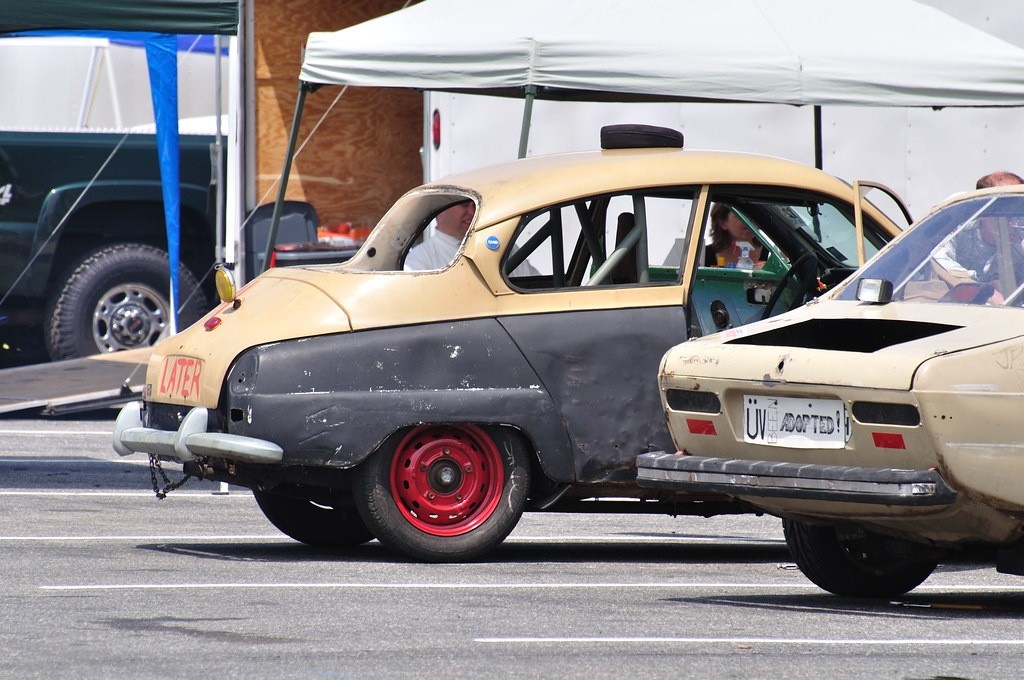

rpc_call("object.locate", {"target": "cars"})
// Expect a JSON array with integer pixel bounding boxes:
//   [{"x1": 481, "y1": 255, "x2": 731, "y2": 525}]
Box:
[
  {"x1": 123, "y1": 148, "x2": 915, "y2": 573},
  {"x1": 637, "y1": 183, "x2": 1024, "y2": 615}
]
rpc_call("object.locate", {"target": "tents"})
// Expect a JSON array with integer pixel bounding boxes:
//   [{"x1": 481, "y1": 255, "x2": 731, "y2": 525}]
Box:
[{"x1": 254, "y1": 0, "x2": 1024, "y2": 392}]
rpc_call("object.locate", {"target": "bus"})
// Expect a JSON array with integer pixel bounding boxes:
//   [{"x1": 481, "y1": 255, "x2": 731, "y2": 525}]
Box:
[{"x1": 0, "y1": 31, "x2": 442, "y2": 372}]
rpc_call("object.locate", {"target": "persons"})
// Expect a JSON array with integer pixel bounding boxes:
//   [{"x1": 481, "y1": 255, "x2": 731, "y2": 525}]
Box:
[
  {"x1": 923, "y1": 171, "x2": 1024, "y2": 306},
  {"x1": 702, "y1": 204, "x2": 768, "y2": 270},
  {"x1": 405, "y1": 194, "x2": 541, "y2": 281}
]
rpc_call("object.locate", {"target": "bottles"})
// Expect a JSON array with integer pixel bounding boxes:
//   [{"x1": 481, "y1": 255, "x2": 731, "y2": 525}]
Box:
[{"x1": 735, "y1": 246, "x2": 755, "y2": 270}]
